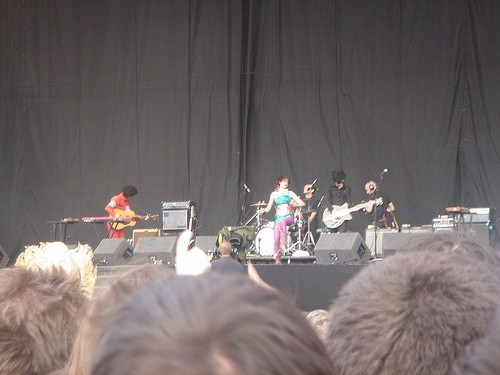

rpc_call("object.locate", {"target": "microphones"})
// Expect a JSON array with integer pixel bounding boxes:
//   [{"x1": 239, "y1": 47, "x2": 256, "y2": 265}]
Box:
[
  {"x1": 242, "y1": 183, "x2": 250, "y2": 192},
  {"x1": 381, "y1": 169, "x2": 388, "y2": 177},
  {"x1": 306, "y1": 179, "x2": 318, "y2": 191}
]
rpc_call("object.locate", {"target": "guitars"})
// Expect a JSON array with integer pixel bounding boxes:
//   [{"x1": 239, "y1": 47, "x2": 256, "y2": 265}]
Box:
[
  {"x1": 322, "y1": 197, "x2": 383, "y2": 229},
  {"x1": 110, "y1": 209, "x2": 159, "y2": 231},
  {"x1": 188, "y1": 205, "x2": 195, "y2": 249}
]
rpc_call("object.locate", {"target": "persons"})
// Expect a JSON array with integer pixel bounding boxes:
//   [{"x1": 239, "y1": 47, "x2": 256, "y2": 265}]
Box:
[
  {"x1": 300, "y1": 184, "x2": 319, "y2": 243},
  {"x1": 105, "y1": 185, "x2": 141, "y2": 239},
  {"x1": 0, "y1": 230, "x2": 500, "y2": 375},
  {"x1": 325, "y1": 169, "x2": 352, "y2": 233},
  {"x1": 260, "y1": 177, "x2": 306, "y2": 265},
  {"x1": 360, "y1": 181, "x2": 395, "y2": 262}
]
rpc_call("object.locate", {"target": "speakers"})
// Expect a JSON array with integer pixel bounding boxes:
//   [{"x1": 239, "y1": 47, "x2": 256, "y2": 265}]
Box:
[
  {"x1": 0, "y1": 246, "x2": 10, "y2": 267},
  {"x1": 132, "y1": 236, "x2": 177, "y2": 266},
  {"x1": 382, "y1": 233, "x2": 427, "y2": 257},
  {"x1": 313, "y1": 232, "x2": 371, "y2": 265},
  {"x1": 455, "y1": 221, "x2": 490, "y2": 247},
  {"x1": 161, "y1": 210, "x2": 190, "y2": 231},
  {"x1": 133, "y1": 229, "x2": 160, "y2": 248},
  {"x1": 93, "y1": 237, "x2": 133, "y2": 266}
]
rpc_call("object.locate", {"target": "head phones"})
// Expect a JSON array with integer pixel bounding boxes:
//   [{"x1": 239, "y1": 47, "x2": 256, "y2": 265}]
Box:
[{"x1": 368, "y1": 182, "x2": 374, "y2": 191}]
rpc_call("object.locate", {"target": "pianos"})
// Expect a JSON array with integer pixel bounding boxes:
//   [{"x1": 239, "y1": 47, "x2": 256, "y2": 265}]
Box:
[{"x1": 62, "y1": 216, "x2": 139, "y2": 221}]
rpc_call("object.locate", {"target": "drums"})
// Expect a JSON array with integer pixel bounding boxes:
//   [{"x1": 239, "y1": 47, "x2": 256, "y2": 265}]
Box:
[{"x1": 252, "y1": 221, "x2": 276, "y2": 257}]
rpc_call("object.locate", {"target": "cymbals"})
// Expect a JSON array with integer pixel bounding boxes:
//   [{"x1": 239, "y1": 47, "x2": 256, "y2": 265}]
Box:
[
  {"x1": 252, "y1": 203, "x2": 271, "y2": 206},
  {"x1": 299, "y1": 209, "x2": 318, "y2": 215}
]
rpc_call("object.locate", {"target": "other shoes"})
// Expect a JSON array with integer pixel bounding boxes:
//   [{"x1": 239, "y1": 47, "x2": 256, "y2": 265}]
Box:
[{"x1": 275, "y1": 256, "x2": 281, "y2": 265}]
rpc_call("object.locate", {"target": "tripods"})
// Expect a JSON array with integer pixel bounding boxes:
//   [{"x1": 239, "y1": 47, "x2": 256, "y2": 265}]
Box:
[{"x1": 283, "y1": 211, "x2": 315, "y2": 255}]
